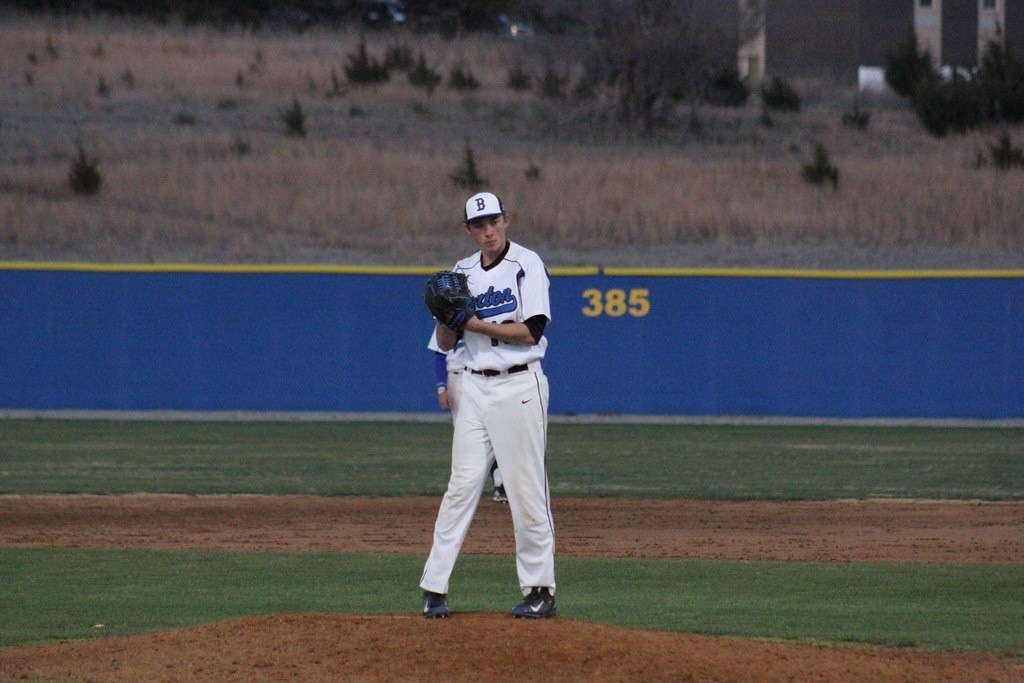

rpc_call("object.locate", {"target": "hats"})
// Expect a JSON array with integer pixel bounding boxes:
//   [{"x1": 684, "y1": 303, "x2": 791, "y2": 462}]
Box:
[{"x1": 464, "y1": 192, "x2": 505, "y2": 224}]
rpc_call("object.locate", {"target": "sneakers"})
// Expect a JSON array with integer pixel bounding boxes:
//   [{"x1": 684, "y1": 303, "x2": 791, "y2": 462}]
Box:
[
  {"x1": 423, "y1": 591, "x2": 450, "y2": 618},
  {"x1": 511, "y1": 587, "x2": 557, "y2": 619},
  {"x1": 492, "y1": 484, "x2": 508, "y2": 503}
]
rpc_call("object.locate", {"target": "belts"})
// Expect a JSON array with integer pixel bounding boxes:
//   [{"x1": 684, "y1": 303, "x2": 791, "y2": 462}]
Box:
[
  {"x1": 464, "y1": 364, "x2": 529, "y2": 376},
  {"x1": 453, "y1": 371, "x2": 459, "y2": 375}
]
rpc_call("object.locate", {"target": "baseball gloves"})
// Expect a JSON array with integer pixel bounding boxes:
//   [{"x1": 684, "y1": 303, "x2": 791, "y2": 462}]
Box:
[{"x1": 421, "y1": 269, "x2": 477, "y2": 333}]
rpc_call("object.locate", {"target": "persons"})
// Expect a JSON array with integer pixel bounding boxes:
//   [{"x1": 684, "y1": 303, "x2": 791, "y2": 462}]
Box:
[
  {"x1": 416, "y1": 192, "x2": 557, "y2": 618},
  {"x1": 426, "y1": 321, "x2": 506, "y2": 503}
]
